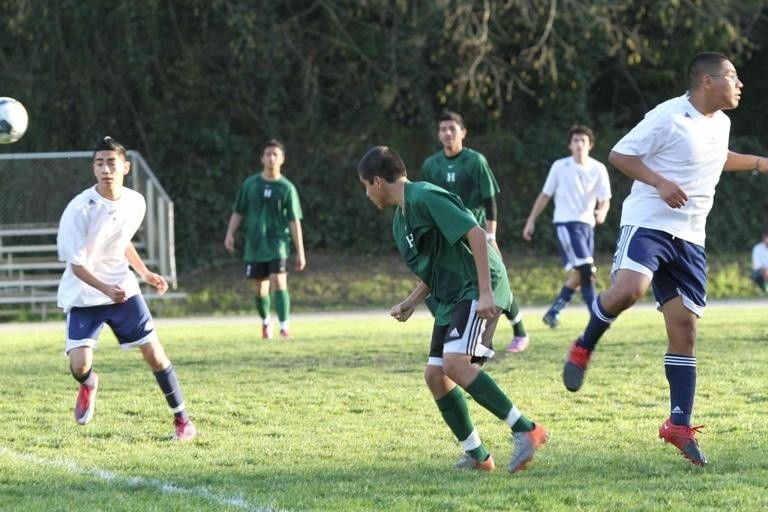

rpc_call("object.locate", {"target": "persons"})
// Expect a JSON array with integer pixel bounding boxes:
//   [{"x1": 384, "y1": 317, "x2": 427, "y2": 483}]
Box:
[
  {"x1": 55, "y1": 136, "x2": 198, "y2": 439},
  {"x1": 522, "y1": 124, "x2": 614, "y2": 326},
  {"x1": 224, "y1": 138, "x2": 307, "y2": 339},
  {"x1": 419, "y1": 109, "x2": 531, "y2": 352},
  {"x1": 356, "y1": 144, "x2": 547, "y2": 475},
  {"x1": 561, "y1": 51, "x2": 768, "y2": 461},
  {"x1": 749, "y1": 228, "x2": 768, "y2": 296}
]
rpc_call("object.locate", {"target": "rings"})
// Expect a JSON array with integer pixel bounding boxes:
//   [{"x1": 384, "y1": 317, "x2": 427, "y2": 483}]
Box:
[
  {"x1": 480, "y1": 311, "x2": 484, "y2": 314},
  {"x1": 119, "y1": 294, "x2": 122, "y2": 298}
]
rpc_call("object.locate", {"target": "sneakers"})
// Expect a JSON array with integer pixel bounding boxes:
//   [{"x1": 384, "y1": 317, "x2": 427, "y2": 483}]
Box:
[
  {"x1": 74, "y1": 372, "x2": 99, "y2": 424},
  {"x1": 564, "y1": 336, "x2": 594, "y2": 391},
  {"x1": 453, "y1": 454, "x2": 495, "y2": 471},
  {"x1": 172, "y1": 415, "x2": 197, "y2": 441},
  {"x1": 659, "y1": 418, "x2": 707, "y2": 467},
  {"x1": 262, "y1": 315, "x2": 272, "y2": 339},
  {"x1": 279, "y1": 328, "x2": 293, "y2": 338},
  {"x1": 542, "y1": 312, "x2": 561, "y2": 329},
  {"x1": 506, "y1": 333, "x2": 530, "y2": 353},
  {"x1": 506, "y1": 421, "x2": 552, "y2": 473}
]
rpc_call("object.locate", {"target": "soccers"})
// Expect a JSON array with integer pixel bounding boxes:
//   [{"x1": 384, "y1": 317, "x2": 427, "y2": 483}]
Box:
[{"x1": 0, "y1": 97, "x2": 29, "y2": 143}]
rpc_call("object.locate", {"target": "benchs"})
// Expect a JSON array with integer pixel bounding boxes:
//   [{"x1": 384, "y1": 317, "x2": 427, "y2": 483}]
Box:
[{"x1": 1, "y1": 146, "x2": 186, "y2": 322}]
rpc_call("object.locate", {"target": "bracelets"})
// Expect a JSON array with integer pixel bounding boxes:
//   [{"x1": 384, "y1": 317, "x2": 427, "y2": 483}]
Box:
[
  {"x1": 485, "y1": 231, "x2": 497, "y2": 241},
  {"x1": 755, "y1": 156, "x2": 762, "y2": 172}
]
